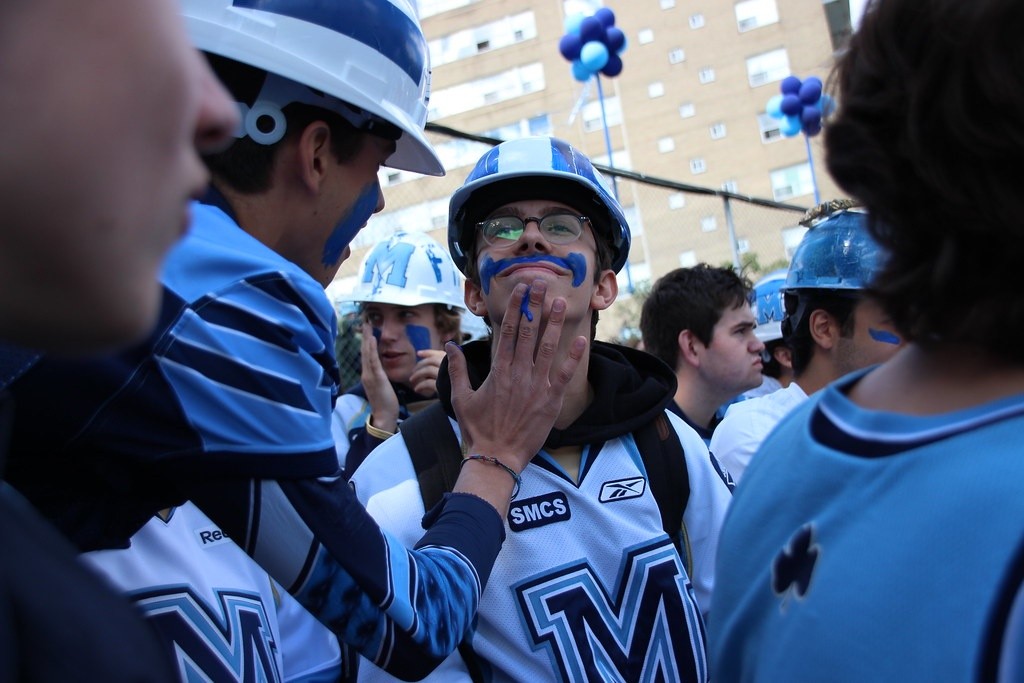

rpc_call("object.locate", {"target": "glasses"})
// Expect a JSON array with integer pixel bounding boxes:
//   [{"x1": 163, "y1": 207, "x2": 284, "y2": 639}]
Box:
[{"x1": 472, "y1": 212, "x2": 605, "y2": 272}]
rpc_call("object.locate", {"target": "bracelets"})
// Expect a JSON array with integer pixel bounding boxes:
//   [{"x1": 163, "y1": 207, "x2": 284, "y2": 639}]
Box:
[{"x1": 460, "y1": 453, "x2": 522, "y2": 502}]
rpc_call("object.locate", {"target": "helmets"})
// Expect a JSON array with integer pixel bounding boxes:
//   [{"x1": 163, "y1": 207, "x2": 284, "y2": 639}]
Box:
[
  {"x1": 746, "y1": 270, "x2": 792, "y2": 343},
  {"x1": 446, "y1": 135, "x2": 631, "y2": 285},
  {"x1": 337, "y1": 231, "x2": 470, "y2": 313},
  {"x1": 181, "y1": 0, "x2": 446, "y2": 178},
  {"x1": 779, "y1": 205, "x2": 895, "y2": 291}
]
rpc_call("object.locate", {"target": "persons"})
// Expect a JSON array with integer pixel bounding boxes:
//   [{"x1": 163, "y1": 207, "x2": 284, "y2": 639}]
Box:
[
  {"x1": 1, "y1": 1, "x2": 590, "y2": 683},
  {"x1": 343, "y1": 136, "x2": 741, "y2": 683},
  {"x1": 639, "y1": 210, "x2": 910, "y2": 632},
  {"x1": 1, "y1": 0, "x2": 242, "y2": 682},
  {"x1": 707, "y1": 0, "x2": 1023, "y2": 683}
]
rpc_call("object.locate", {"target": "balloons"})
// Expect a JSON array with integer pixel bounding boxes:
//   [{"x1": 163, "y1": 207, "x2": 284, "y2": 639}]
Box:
[
  {"x1": 766, "y1": 74, "x2": 836, "y2": 138},
  {"x1": 554, "y1": 6, "x2": 629, "y2": 86}
]
